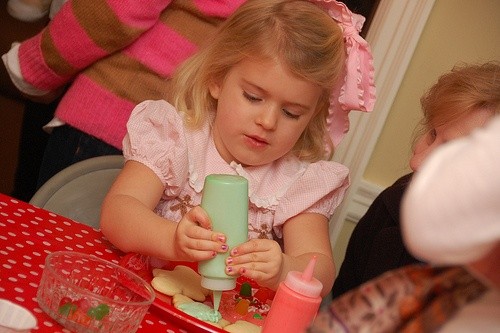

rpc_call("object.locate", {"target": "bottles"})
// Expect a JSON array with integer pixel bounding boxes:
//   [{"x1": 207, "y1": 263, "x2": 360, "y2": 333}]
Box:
[
  {"x1": 197, "y1": 174, "x2": 249, "y2": 311},
  {"x1": 260, "y1": 255, "x2": 323, "y2": 333}
]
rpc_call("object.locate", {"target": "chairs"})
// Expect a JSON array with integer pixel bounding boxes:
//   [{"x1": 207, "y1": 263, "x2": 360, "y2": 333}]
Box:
[{"x1": 30, "y1": 154, "x2": 126, "y2": 230}]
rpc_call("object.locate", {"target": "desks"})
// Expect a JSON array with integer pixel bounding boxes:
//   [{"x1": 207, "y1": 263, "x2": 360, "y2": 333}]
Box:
[{"x1": 0, "y1": 192, "x2": 277, "y2": 333}]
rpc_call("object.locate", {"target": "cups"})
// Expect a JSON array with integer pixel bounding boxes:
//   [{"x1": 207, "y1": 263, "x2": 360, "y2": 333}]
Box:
[{"x1": 0, "y1": 299, "x2": 37, "y2": 333}]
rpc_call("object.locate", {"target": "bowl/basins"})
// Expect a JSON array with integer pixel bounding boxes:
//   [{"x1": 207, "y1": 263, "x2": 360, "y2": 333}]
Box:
[{"x1": 35, "y1": 251, "x2": 156, "y2": 333}]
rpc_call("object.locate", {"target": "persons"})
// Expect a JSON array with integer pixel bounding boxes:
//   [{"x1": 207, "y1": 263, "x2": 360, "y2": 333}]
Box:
[
  {"x1": 1, "y1": 0, "x2": 251, "y2": 205},
  {"x1": 98, "y1": 0, "x2": 350, "y2": 300},
  {"x1": 302, "y1": 60, "x2": 500, "y2": 333}
]
